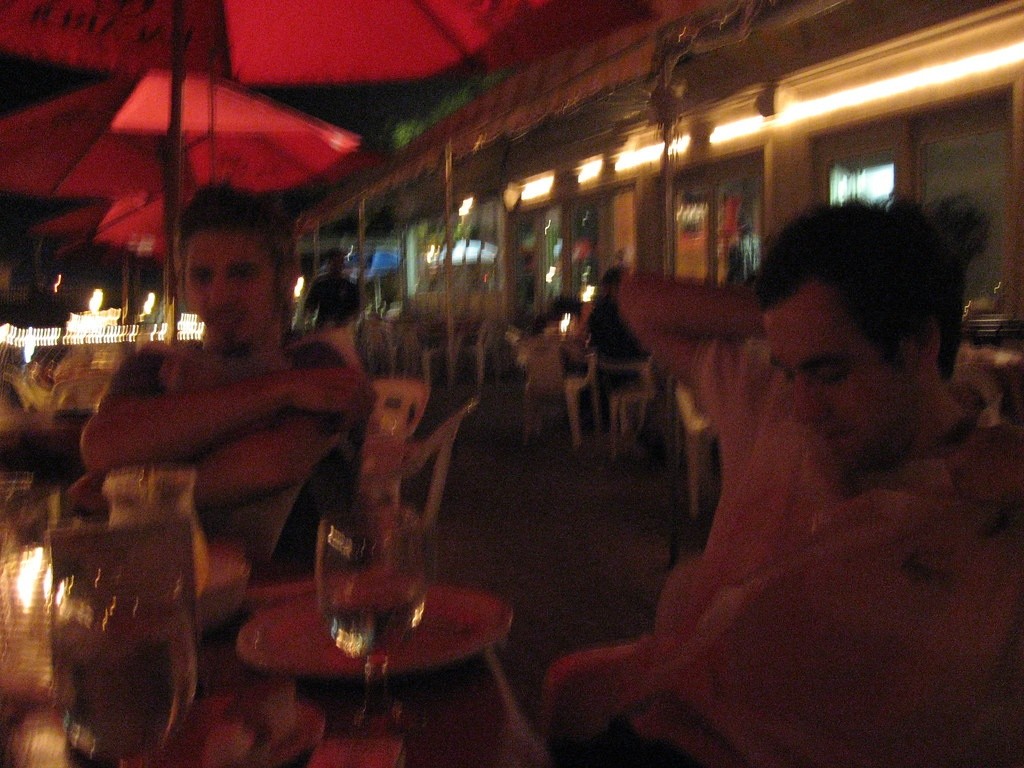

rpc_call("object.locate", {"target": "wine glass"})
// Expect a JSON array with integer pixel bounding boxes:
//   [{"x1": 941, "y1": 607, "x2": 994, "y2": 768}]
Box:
[{"x1": 313, "y1": 513, "x2": 429, "y2": 738}]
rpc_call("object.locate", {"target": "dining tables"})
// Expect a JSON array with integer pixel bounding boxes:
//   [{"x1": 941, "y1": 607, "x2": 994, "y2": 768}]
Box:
[{"x1": 1, "y1": 577, "x2": 553, "y2": 768}]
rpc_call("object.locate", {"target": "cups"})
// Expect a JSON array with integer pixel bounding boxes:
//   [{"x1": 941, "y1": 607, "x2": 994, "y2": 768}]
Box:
[
  {"x1": 50, "y1": 567, "x2": 192, "y2": 764},
  {"x1": 105, "y1": 467, "x2": 211, "y2": 610}
]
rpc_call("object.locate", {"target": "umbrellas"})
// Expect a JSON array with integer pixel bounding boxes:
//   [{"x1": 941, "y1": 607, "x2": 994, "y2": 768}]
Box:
[
  {"x1": 0, "y1": 0, "x2": 547, "y2": 80},
  {"x1": 31, "y1": 190, "x2": 192, "y2": 257},
  {"x1": 0, "y1": 66, "x2": 360, "y2": 203}
]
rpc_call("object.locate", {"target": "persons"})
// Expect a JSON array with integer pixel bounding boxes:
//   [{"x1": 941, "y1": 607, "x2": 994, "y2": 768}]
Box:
[
  {"x1": 68, "y1": 178, "x2": 379, "y2": 570},
  {"x1": 578, "y1": 266, "x2": 642, "y2": 432},
  {"x1": 292, "y1": 247, "x2": 364, "y2": 331},
  {"x1": 543, "y1": 198, "x2": 1024, "y2": 767}
]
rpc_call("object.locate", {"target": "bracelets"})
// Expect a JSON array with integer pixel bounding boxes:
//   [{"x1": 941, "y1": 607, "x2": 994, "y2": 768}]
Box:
[{"x1": 135, "y1": 464, "x2": 151, "y2": 505}]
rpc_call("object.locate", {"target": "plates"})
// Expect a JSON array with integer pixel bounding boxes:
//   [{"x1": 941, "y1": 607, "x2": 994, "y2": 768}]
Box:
[{"x1": 235, "y1": 586, "x2": 514, "y2": 683}]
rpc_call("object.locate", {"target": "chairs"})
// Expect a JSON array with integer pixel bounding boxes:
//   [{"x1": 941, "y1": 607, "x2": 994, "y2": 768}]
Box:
[
  {"x1": 2, "y1": 295, "x2": 723, "y2": 554},
  {"x1": 535, "y1": 623, "x2": 679, "y2": 768}
]
